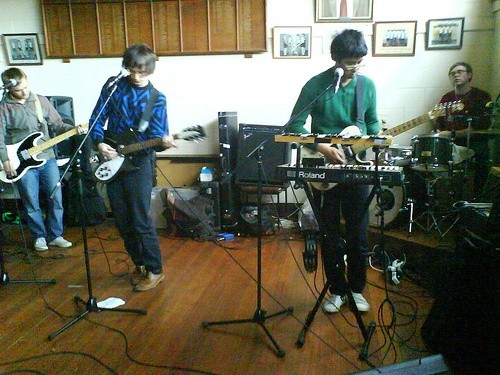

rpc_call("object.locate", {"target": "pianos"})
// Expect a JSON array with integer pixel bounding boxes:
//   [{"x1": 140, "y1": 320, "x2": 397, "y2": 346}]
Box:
[{"x1": 276, "y1": 163, "x2": 404, "y2": 346}]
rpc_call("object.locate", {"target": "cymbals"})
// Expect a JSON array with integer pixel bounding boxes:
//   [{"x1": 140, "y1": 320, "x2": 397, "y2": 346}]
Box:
[{"x1": 472, "y1": 129, "x2": 500, "y2": 138}]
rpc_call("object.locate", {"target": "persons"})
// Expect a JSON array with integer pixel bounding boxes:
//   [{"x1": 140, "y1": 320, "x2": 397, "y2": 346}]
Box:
[
  {"x1": 88, "y1": 43, "x2": 176, "y2": 291},
  {"x1": 281, "y1": 32, "x2": 307, "y2": 56},
  {"x1": 384, "y1": 28, "x2": 409, "y2": 48},
  {"x1": 437, "y1": 24, "x2": 454, "y2": 45},
  {"x1": 435, "y1": 62, "x2": 493, "y2": 195},
  {"x1": 322, "y1": 0, "x2": 369, "y2": 17},
  {"x1": 1, "y1": 68, "x2": 88, "y2": 254},
  {"x1": 14, "y1": 40, "x2": 36, "y2": 59},
  {"x1": 284, "y1": 29, "x2": 394, "y2": 313}
]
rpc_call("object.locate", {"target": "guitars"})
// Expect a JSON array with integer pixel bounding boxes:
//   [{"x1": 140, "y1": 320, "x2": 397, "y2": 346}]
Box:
[
  {"x1": 79, "y1": 125, "x2": 204, "y2": 184},
  {"x1": 297, "y1": 100, "x2": 464, "y2": 192},
  {"x1": 0, "y1": 125, "x2": 88, "y2": 183}
]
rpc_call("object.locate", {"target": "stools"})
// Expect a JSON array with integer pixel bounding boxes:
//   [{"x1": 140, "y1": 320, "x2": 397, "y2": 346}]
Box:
[{"x1": 238, "y1": 185, "x2": 287, "y2": 234}]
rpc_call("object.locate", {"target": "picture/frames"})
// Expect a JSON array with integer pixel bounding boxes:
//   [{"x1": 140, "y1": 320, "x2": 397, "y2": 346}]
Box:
[
  {"x1": 424, "y1": 16, "x2": 464, "y2": 51},
  {"x1": 372, "y1": 21, "x2": 417, "y2": 57},
  {"x1": 314, "y1": 1, "x2": 374, "y2": 23},
  {"x1": 2, "y1": 32, "x2": 44, "y2": 66},
  {"x1": 271, "y1": 26, "x2": 313, "y2": 59}
]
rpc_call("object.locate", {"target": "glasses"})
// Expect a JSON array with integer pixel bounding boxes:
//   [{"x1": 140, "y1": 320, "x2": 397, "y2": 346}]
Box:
[
  {"x1": 339, "y1": 58, "x2": 365, "y2": 70},
  {"x1": 448, "y1": 70, "x2": 470, "y2": 77}
]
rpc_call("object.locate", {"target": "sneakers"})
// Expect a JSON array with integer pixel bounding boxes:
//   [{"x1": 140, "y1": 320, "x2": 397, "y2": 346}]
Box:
[
  {"x1": 323, "y1": 294, "x2": 347, "y2": 312},
  {"x1": 48, "y1": 236, "x2": 72, "y2": 247},
  {"x1": 352, "y1": 291, "x2": 370, "y2": 311},
  {"x1": 34, "y1": 237, "x2": 48, "y2": 251}
]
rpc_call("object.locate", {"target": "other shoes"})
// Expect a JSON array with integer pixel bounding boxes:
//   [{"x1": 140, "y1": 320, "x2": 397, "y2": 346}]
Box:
[
  {"x1": 130, "y1": 270, "x2": 148, "y2": 284},
  {"x1": 135, "y1": 271, "x2": 165, "y2": 291}
]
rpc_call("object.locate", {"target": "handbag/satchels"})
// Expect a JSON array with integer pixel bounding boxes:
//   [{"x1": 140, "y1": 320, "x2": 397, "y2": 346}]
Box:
[{"x1": 160, "y1": 188, "x2": 215, "y2": 238}]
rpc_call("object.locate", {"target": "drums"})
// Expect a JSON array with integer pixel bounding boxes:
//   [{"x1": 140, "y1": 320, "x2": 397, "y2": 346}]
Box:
[
  {"x1": 365, "y1": 184, "x2": 415, "y2": 227},
  {"x1": 375, "y1": 145, "x2": 410, "y2": 167},
  {"x1": 409, "y1": 135, "x2": 454, "y2": 171}
]
